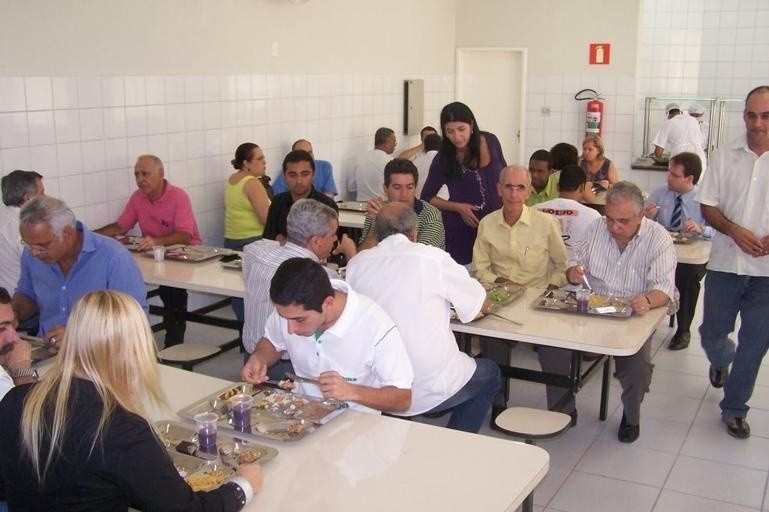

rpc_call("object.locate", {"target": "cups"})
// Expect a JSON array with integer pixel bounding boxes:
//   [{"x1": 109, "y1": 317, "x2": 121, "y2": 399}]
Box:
[
  {"x1": 153, "y1": 262, "x2": 165, "y2": 277},
  {"x1": 575, "y1": 289, "x2": 591, "y2": 310},
  {"x1": 230, "y1": 394, "x2": 253, "y2": 429},
  {"x1": 194, "y1": 412, "x2": 219, "y2": 453},
  {"x1": 152, "y1": 244, "x2": 165, "y2": 262}
]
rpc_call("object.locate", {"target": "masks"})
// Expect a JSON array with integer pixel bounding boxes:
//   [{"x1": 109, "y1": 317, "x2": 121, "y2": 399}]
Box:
[{"x1": 694, "y1": 114, "x2": 706, "y2": 122}]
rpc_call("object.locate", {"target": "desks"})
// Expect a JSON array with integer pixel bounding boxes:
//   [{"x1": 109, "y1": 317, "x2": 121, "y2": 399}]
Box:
[
  {"x1": 17, "y1": 335, "x2": 550, "y2": 510},
  {"x1": 122, "y1": 244, "x2": 669, "y2": 419},
  {"x1": 335, "y1": 201, "x2": 713, "y2": 264}
]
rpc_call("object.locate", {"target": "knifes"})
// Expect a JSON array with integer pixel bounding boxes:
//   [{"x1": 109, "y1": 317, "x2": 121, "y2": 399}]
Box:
[{"x1": 285, "y1": 371, "x2": 320, "y2": 385}]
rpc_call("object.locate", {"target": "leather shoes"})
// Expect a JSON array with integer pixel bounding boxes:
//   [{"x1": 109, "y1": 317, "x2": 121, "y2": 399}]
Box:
[
  {"x1": 490, "y1": 406, "x2": 508, "y2": 431},
  {"x1": 668, "y1": 329, "x2": 692, "y2": 350},
  {"x1": 582, "y1": 351, "x2": 603, "y2": 361},
  {"x1": 618, "y1": 409, "x2": 640, "y2": 444}
]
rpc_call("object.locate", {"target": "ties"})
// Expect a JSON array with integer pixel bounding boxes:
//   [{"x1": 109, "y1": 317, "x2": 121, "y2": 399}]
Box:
[{"x1": 670, "y1": 195, "x2": 683, "y2": 232}]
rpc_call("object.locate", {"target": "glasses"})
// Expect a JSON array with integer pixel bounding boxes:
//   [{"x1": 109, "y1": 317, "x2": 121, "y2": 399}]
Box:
[
  {"x1": 20, "y1": 231, "x2": 57, "y2": 251},
  {"x1": 498, "y1": 181, "x2": 526, "y2": 192}
]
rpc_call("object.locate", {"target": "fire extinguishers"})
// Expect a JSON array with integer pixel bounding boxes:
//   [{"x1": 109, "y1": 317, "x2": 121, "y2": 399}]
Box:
[{"x1": 574, "y1": 88, "x2": 606, "y2": 140}]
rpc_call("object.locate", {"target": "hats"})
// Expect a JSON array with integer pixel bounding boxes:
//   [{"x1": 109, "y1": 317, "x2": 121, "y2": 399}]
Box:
[
  {"x1": 665, "y1": 102, "x2": 680, "y2": 113},
  {"x1": 688, "y1": 103, "x2": 706, "y2": 114}
]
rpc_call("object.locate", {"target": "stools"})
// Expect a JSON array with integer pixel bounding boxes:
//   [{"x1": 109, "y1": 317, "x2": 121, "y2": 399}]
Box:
[
  {"x1": 156, "y1": 341, "x2": 222, "y2": 372},
  {"x1": 495, "y1": 406, "x2": 573, "y2": 447}
]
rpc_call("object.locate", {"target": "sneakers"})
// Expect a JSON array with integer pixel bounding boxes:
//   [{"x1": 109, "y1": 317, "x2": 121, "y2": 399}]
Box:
[
  {"x1": 159, "y1": 346, "x2": 169, "y2": 364},
  {"x1": 721, "y1": 410, "x2": 751, "y2": 439},
  {"x1": 568, "y1": 408, "x2": 578, "y2": 427},
  {"x1": 709, "y1": 338, "x2": 735, "y2": 388}
]
